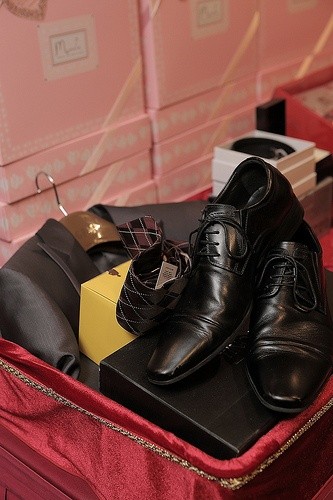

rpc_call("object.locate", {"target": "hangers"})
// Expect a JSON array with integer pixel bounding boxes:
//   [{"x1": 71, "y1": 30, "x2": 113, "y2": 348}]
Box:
[{"x1": 34, "y1": 171, "x2": 122, "y2": 252}]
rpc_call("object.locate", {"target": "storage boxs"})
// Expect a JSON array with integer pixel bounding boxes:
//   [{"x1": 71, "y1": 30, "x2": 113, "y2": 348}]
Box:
[
  {"x1": 0, "y1": 0, "x2": 145, "y2": 166},
  {"x1": 257, "y1": 53, "x2": 333, "y2": 106},
  {"x1": 0, "y1": 149, "x2": 152, "y2": 243},
  {"x1": 0, "y1": 113, "x2": 152, "y2": 204},
  {"x1": 213, "y1": 129, "x2": 317, "y2": 172},
  {"x1": 78, "y1": 260, "x2": 138, "y2": 366},
  {"x1": 98, "y1": 267, "x2": 333, "y2": 460},
  {"x1": 212, "y1": 174, "x2": 316, "y2": 201},
  {"x1": 145, "y1": 72, "x2": 259, "y2": 144},
  {"x1": 0, "y1": 180, "x2": 158, "y2": 267},
  {"x1": 138, "y1": 0, "x2": 258, "y2": 110},
  {"x1": 272, "y1": 64, "x2": 333, "y2": 154},
  {"x1": 153, "y1": 154, "x2": 213, "y2": 204},
  {"x1": 258, "y1": 0, "x2": 333, "y2": 72},
  {"x1": 210, "y1": 156, "x2": 315, "y2": 187},
  {"x1": 151, "y1": 106, "x2": 257, "y2": 177}
]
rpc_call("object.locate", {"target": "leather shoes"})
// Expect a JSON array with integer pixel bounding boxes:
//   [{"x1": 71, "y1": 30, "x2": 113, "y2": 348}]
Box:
[
  {"x1": 244, "y1": 219, "x2": 333, "y2": 414},
  {"x1": 146, "y1": 156, "x2": 305, "y2": 386}
]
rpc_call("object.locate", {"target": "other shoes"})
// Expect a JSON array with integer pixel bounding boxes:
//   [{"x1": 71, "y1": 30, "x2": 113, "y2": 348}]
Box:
[
  {"x1": 117, "y1": 216, "x2": 165, "y2": 259},
  {"x1": 116, "y1": 238, "x2": 194, "y2": 337}
]
